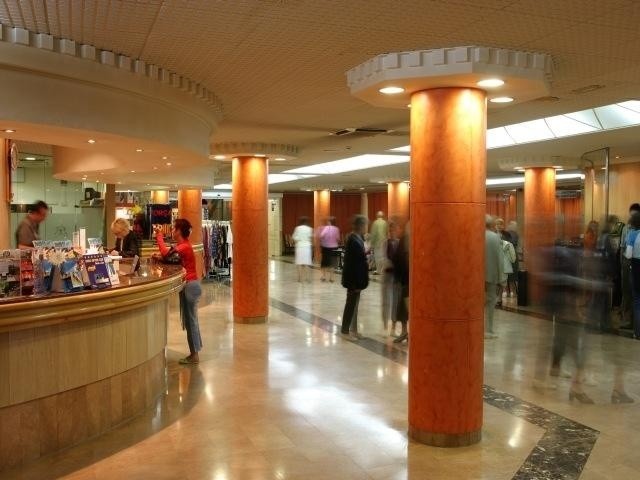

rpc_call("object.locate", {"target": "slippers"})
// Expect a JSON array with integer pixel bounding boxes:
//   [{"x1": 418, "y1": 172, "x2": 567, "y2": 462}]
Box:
[{"x1": 179, "y1": 357, "x2": 200, "y2": 365}]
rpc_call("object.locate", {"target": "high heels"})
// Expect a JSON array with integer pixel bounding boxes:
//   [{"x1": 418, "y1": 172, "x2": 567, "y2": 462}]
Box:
[
  {"x1": 393, "y1": 333, "x2": 408, "y2": 344},
  {"x1": 611, "y1": 390, "x2": 636, "y2": 405},
  {"x1": 568, "y1": 388, "x2": 596, "y2": 405}
]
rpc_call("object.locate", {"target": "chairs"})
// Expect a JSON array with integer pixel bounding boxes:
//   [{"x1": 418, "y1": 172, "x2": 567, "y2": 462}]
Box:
[{"x1": 283, "y1": 232, "x2": 295, "y2": 255}]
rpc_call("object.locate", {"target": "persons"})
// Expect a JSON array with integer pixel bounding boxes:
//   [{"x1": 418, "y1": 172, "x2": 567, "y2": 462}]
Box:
[
  {"x1": 107, "y1": 217, "x2": 140, "y2": 263},
  {"x1": 153, "y1": 217, "x2": 203, "y2": 364},
  {"x1": 15, "y1": 200, "x2": 48, "y2": 249},
  {"x1": 484, "y1": 204, "x2": 639, "y2": 401},
  {"x1": 292, "y1": 210, "x2": 410, "y2": 342}
]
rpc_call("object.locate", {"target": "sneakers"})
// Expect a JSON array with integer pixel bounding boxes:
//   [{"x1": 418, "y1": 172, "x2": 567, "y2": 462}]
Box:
[{"x1": 548, "y1": 367, "x2": 573, "y2": 379}]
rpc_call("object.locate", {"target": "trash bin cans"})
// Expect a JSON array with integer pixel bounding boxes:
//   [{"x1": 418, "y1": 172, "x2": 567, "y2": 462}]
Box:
[{"x1": 518, "y1": 270, "x2": 528, "y2": 306}]
